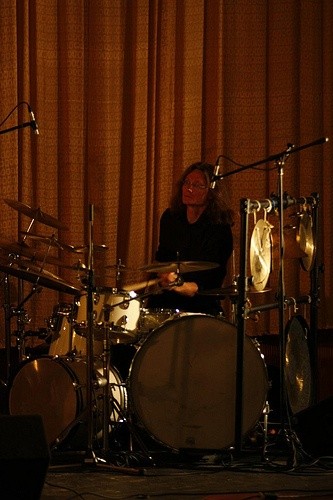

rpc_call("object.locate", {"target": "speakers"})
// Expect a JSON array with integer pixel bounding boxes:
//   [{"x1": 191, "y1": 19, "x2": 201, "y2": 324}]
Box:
[{"x1": 0, "y1": 415, "x2": 48, "y2": 500}]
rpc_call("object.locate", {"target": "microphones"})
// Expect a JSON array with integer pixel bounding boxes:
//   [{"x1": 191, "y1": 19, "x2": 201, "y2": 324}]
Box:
[
  {"x1": 28, "y1": 106, "x2": 39, "y2": 135},
  {"x1": 210, "y1": 159, "x2": 220, "y2": 189}
]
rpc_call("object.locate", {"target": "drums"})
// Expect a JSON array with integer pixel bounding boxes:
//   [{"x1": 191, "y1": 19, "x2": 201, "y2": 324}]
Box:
[
  {"x1": 249, "y1": 218, "x2": 281, "y2": 292},
  {"x1": 296, "y1": 209, "x2": 315, "y2": 273},
  {"x1": 280, "y1": 314, "x2": 313, "y2": 414},
  {"x1": 72, "y1": 284, "x2": 142, "y2": 343},
  {"x1": 48, "y1": 301, "x2": 110, "y2": 357},
  {"x1": 126, "y1": 310, "x2": 269, "y2": 456},
  {"x1": 5, "y1": 352, "x2": 129, "y2": 458}
]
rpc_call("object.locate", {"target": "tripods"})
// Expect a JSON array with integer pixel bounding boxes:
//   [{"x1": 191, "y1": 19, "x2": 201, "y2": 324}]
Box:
[
  {"x1": 47, "y1": 202, "x2": 145, "y2": 475},
  {"x1": 213, "y1": 137, "x2": 328, "y2": 472}
]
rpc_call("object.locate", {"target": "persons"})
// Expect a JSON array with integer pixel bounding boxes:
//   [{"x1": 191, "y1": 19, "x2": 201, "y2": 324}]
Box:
[{"x1": 141, "y1": 162, "x2": 236, "y2": 315}]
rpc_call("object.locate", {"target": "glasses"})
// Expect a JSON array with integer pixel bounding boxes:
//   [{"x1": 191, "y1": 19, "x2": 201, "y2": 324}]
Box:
[{"x1": 182, "y1": 180, "x2": 207, "y2": 190}]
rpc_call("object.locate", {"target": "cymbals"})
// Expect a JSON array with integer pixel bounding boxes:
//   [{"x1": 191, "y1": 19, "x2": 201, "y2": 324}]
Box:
[
  {"x1": 197, "y1": 283, "x2": 275, "y2": 299},
  {"x1": 0, "y1": 197, "x2": 81, "y2": 296},
  {"x1": 137, "y1": 260, "x2": 220, "y2": 275}
]
